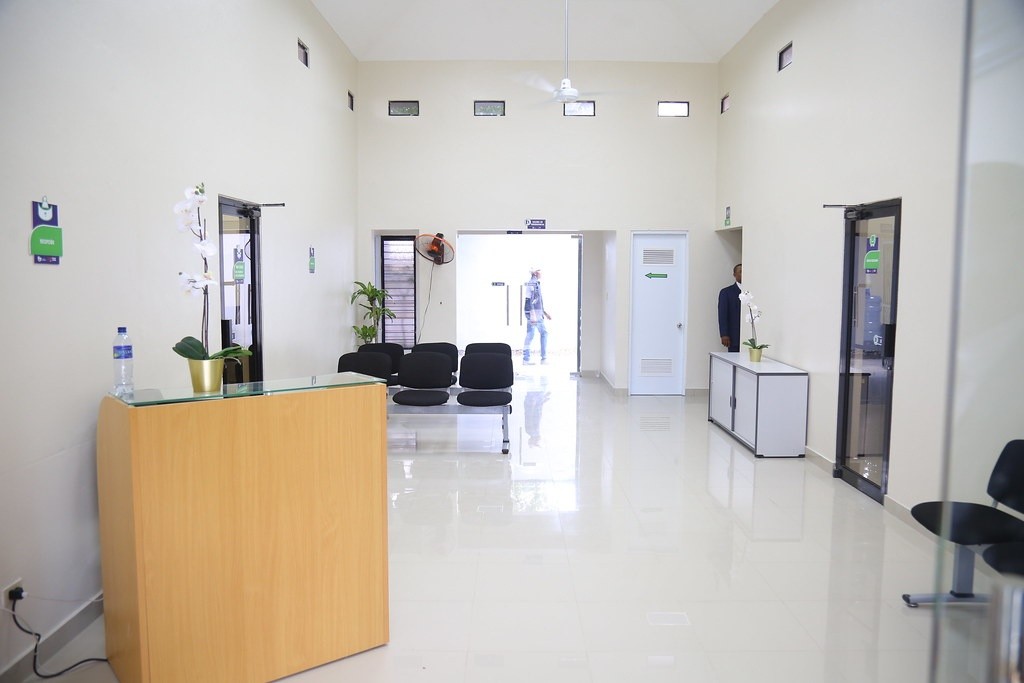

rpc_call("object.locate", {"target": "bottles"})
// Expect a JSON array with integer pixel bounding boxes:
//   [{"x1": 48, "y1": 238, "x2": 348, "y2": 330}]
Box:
[
  {"x1": 112, "y1": 327, "x2": 133, "y2": 397},
  {"x1": 865, "y1": 289, "x2": 881, "y2": 342}
]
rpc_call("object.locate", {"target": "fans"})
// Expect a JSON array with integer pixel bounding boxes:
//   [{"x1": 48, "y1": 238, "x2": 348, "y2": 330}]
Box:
[
  {"x1": 413, "y1": 232, "x2": 455, "y2": 264},
  {"x1": 497, "y1": 0, "x2": 640, "y2": 103}
]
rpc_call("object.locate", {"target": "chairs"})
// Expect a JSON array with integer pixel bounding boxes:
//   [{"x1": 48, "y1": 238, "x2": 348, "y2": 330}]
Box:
[
  {"x1": 337, "y1": 342, "x2": 514, "y2": 454},
  {"x1": 902, "y1": 440, "x2": 1024, "y2": 683}
]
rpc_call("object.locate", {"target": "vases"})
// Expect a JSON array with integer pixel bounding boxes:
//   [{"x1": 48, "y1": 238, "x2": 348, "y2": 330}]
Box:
[
  {"x1": 749, "y1": 348, "x2": 762, "y2": 362},
  {"x1": 188, "y1": 358, "x2": 224, "y2": 392}
]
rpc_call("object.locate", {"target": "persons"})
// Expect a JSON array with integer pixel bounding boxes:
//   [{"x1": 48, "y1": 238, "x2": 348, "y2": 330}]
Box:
[
  {"x1": 717, "y1": 264, "x2": 742, "y2": 353},
  {"x1": 523, "y1": 267, "x2": 553, "y2": 365}
]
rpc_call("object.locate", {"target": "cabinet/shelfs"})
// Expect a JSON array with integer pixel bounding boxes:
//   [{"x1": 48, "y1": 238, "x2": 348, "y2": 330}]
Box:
[
  {"x1": 95, "y1": 371, "x2": 390, "y2": 683},
  {"x1": 708, "y1": 351, "x2": 809, "y2": 458}
]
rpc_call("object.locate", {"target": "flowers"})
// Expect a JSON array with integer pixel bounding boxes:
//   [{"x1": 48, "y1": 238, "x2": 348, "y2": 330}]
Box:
[
  {"x1": 171, "y1": 182, "x2": 252, "y2": 365},
  {"x1": 738, "y1": 290, "x2": 770, "y2": 349}
]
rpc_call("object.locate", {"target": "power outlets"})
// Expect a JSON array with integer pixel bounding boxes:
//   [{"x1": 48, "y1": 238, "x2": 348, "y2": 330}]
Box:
[{"x1": 3, "y1": 578, "x2": 25, "y2": 610}]
